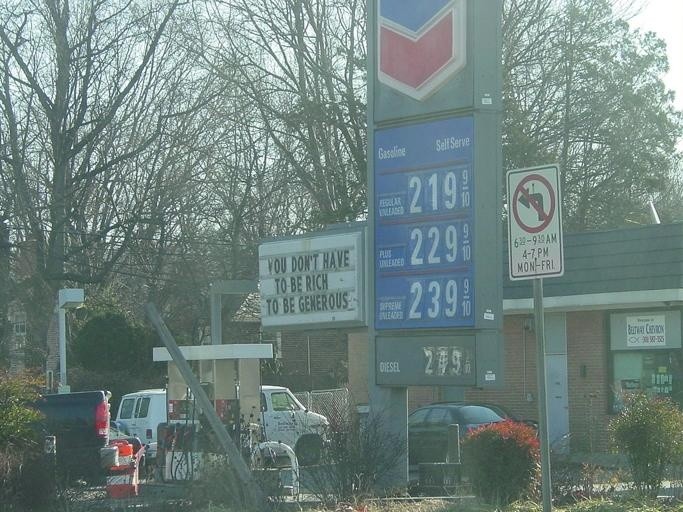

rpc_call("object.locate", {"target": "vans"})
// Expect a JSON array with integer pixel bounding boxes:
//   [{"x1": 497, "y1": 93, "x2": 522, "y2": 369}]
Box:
[{"x1": 110, "y1": 384, "x2": 329, "y2": 481}]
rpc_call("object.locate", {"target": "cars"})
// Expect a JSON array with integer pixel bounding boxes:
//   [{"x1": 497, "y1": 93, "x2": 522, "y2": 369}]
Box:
[{"x1": 407, "y1": 401, "x2": 538, "y2": 469}]
